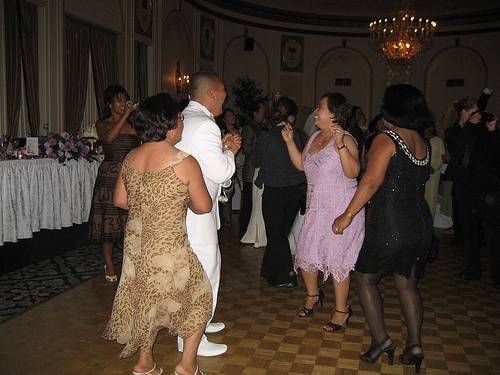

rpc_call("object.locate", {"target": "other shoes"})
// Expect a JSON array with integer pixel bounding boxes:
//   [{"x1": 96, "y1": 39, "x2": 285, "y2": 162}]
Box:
[
  {"x1": 458, "y1": 272, "x2": 481, "y2": 280},
  {"x1": 178, "y1": 333, "x2": 228, "y2": 357},
  {"x1": 204, "y1": 322, "x2": 225, "y2": 333},
  {"x1": 254, "y1": 242, "x2": 265, "y2": 249}
]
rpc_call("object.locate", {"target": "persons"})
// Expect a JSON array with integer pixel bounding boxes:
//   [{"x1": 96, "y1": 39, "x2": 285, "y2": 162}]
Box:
[
  {"x1": 331, "y1": 82, "x2": 435, "y2": 374},
  {"x1": 100, "y1": 93, "x2": 213, "y2": 375},
  {"x1": 86, "y1": 84, "x2": 142, "y2": 282},
  {"x1": 281, "y1": 93, "x2": 365, "y2": 331},
  {"x1": 173, "y1": 72, "x2": 243, "y2": 355},
  {"x1": 444, "y1": 88, "x2": 500, "y2": 289},
  {"x1": 217, "y1": 96, "x2": 451, "y2": 287}
]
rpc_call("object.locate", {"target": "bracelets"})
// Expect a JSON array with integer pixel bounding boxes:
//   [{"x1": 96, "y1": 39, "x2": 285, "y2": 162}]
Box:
[
  {"x1": 338, "y1": 144, "x2": 346, "y2": 150},
  {"x1": 345, "y1": 210, "x2": 354, "y2": 219},
  {"x1": 223, "y1": 145, "x2": 229, "y2": 149}
]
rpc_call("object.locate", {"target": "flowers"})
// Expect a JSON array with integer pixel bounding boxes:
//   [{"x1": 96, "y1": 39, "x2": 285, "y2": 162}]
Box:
[{"x1": 1, "y1": 129, "x2": 103, "y2": 163}]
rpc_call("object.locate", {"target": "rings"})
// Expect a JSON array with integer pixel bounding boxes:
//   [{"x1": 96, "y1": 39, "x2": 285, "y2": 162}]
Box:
[
  {"x1": 336, "y1": 133, "x2": 338, "y2": 136},
  {"x1": 335, "y1": 227, "x2": 338, "y2": 231},
  {"x1": 338, "y1": 130, "x2": 340, "y2": 133}
]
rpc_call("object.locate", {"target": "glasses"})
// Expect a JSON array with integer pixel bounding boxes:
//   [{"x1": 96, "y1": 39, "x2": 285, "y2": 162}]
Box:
[{"x1": 177, "y1": 114, "x2": 184, "y2": 121}]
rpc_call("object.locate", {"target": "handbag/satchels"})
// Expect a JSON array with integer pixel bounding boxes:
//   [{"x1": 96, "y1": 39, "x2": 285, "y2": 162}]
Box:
[{"x1": 438, "y1": 148, "x2": 471, "y2": 182}]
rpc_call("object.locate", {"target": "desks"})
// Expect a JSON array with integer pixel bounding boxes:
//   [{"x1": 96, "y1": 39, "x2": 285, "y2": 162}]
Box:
[{"x1": 0, "y1": 154, "x2": 104, "y2": 276}]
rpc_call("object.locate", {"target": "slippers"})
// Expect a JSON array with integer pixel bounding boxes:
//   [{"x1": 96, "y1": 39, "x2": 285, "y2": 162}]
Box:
[
  {"x1": 175, "y1": 364, "x2": 208, "y2": 375},
  {"x1": 132, "y1": 362, "x2": 164, "y2": 375}
]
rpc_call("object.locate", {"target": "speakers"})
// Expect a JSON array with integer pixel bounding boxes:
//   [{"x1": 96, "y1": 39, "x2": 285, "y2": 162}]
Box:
[{"x1": 244, "y1": 38, "x2": 254, "y2": 50}]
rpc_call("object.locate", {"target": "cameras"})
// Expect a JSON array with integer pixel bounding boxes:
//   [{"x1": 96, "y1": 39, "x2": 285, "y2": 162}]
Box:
[
  {"x1": 128, "y1": 103, "x2": 140, "y2": 110},
  {"x1": 472, "y1": 89, "x2": 494, "y2": 122}
]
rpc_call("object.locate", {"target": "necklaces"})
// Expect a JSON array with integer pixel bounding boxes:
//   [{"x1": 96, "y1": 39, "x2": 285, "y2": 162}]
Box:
[{"x1": 318, "y1": 138, "x2": 325, "y2": 146}]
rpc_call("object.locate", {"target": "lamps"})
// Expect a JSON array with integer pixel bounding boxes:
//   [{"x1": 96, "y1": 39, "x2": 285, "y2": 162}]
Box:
[
  {"x1": 369, "y1": 0, "x2": 437, "y2": 65},
  {"x1": 176, "y1": 62, "x2": 190, "y2": 97}
]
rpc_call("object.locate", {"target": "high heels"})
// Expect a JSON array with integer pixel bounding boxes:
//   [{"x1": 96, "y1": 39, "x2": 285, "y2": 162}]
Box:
[
  {"x1": 323, "y1": 304, "x2": 352, "y2": 331},
  {"x1": 359, "y1": 337, "x2": 395, "y2": 366},
  {"x1": 299, "y1": 290, "x2": 324, "y2": 317},
  {"x1": 104, "y1": 265, "x2": 117, "y2": 282},
  {"x1": 399, "y1": 344, "x2": 424, "y2": 374}
]
